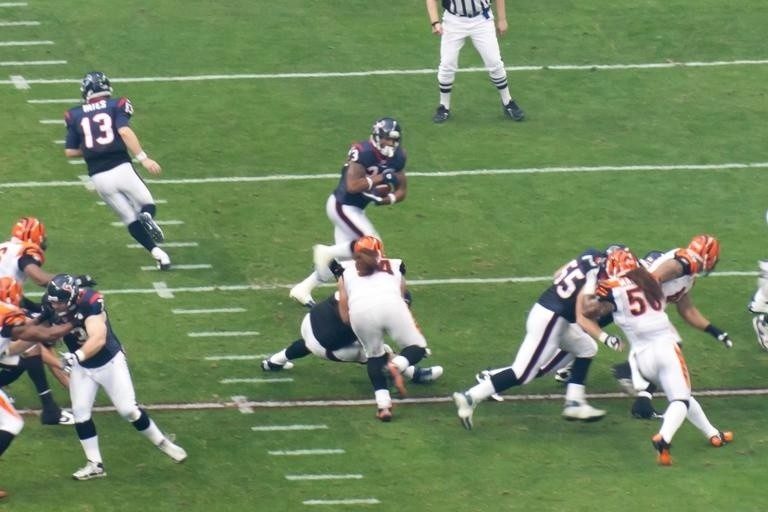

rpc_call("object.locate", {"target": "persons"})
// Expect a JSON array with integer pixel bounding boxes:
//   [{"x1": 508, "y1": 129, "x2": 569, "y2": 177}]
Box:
[
  {"x1": 611, "y1": 232, "x2": 733, "y2": 419},
  {"x1": 289, "y1": 116, "x2": 407, "y2": 308},
  {"x1": 261, "y1": 241, "x2": 444, "y2": 383},
  {"x1": 336, "y1": 235, "x2": 427, "y2": 421},
  {"x1": 0, "y1": 217, "x2": 187, "y2": 496},
  {"x1": 581, "y1": 248, "x2": 732, "y2": 466},
  {"x1": 748, "y1": 260, "x2": 768, "y2": 354},
  {"x1": 454, "y1": 245, "x2": 666, "y2": 431},
  {"x1": 63, "y1": 71, "x2": 172, "y2": 272},
  {"x1": 424, "y1": 0, "x2": 525, "y2": 123}
]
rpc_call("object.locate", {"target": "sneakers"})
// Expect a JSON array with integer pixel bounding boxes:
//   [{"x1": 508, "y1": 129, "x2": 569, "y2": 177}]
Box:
[
  {"x1": 453, "y1": 391, "x2": 475, "y2": 430},
  {"x1": 710, "y1": 431, "x2": 733, "y2": 447},
  {"x1": 138, "y1": 212, "x2": 164, "y2": 243},
  {"x1": 159, "y1": 257, "x2": 171, "y2": 269},
  {"x1": 503, "y1": 100, "x2": 523, "y2": 121},
  {"x1": 157, "y1": 440, "x2": 187, "y2": 462},
  {"x1": 289, "y1": 287, "x2": 316, "y2": 308},
  {"x1": 752, "y1": 314, "x2": 768, "y2": 350},
  {"x1": 261, "y1": 360, "x2": 294, "y2": 371},
  {"x1": 476, "y1": 369, "x2": 505, "y2": 401},
  {"x1": 556, "y1": 362, "x2": 662, "y2": 420},
  {"x1": 41, "y1": 409, "x2": 75, "y2": 426},
  {"x1": 313, "y1": 244, "x2": 335, "y2": 282},
  {"x1": 72, "y1": 459, "x2": 106, "y2": 480},
  {"x1": 433, "y1": 105, "x2": 448, "y2": 123},
  {"x1": 375, "y1": 360, "x2": 444, "y2": 420},
  {"x1": 652, "y1": 434, "x2": 672, "y2": 465}
]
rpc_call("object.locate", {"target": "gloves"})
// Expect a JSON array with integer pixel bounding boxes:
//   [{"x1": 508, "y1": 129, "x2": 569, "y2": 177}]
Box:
[
  {"x1": 599, "y1": 332, "x2": 623, "y2": 351},
  {"x1": 584, "y1": 269, "x2": 599, "y2": 294},
  {"x1": 57, "y1": 351, "x2": 77, "y2": 367},
  {"x1": 705, "y1": 323, "x2": 734, "y2": 348}
]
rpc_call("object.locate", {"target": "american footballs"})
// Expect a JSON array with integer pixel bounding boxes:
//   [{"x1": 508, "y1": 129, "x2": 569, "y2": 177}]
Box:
[{"x1": 374, "y1": 183, "x2": 392, "y2": 196}]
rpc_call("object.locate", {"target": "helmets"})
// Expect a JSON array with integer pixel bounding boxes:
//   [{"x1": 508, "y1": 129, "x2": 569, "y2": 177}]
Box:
[
  {"x1": 605, "y1": 247, "x2": 637, "y2": 279},
  {"x1": 80, "y1": 71, "x2": 114, "y2": 103},
  {"x1": 12, "y1": 217, "x2": 48, "y2": 250},
  {"x1": 47, "y1": 274, "x2": 79, "y2": 318},
  {"x1": 688, "y1": 235, "x2": 719, "y2": 276},
  {"x1": 354, "y1": 236, "x2": 381, "y2": 252},
  {"x1": 370, "y1": 118, "x2": 401, "y2": 157}
]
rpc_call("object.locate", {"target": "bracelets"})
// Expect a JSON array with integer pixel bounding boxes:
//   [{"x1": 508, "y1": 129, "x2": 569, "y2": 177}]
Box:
[
  {"x1": 599, "y1": 331, "x2": 609, "y2": 342},
  {"x1": 431, "y1": 21, "x2": 440, "y2": 27},
  {"x1": 703, "y1": 322, "x2": 725, "y2": 339},
  {"x1": 386, "y1": 192, "x2": 397, "y2": 206},
  {"x1": 366, "y1": 175, "x2": 374, "y2": 193},
  {"x1": 132, "y1": 151, "x2": 148, "y2": 164}
]
